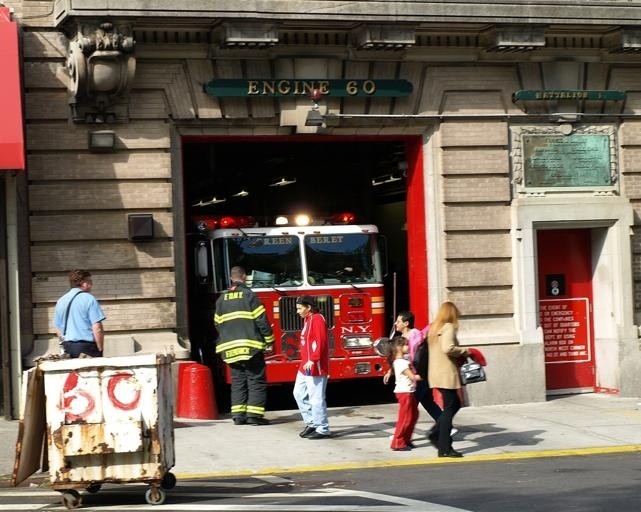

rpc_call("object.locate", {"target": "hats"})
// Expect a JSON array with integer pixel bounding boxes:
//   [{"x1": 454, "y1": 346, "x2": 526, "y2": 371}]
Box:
[{"x1": 297, "y1": 295, "x2": 318, "y2": 306}]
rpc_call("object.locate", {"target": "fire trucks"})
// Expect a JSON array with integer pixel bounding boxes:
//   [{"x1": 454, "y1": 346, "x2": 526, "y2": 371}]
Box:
[{"x1": 193, "y1": 209, "x2": 390, "y2": 385}]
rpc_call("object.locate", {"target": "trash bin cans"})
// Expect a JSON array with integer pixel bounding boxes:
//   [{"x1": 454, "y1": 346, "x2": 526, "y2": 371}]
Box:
[{"x1": 12, "y1": 354, "x2": 176, "y2": 509}]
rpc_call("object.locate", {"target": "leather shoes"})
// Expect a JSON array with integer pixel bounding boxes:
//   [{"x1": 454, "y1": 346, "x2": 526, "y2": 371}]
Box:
[
  {"x1": 247, "y1": 416, "x2": 271, "y2": 424},
  {"x1": 427, "y1": 430, "x2": 438, "y2": 450},
  {"x1": 235, "y1": 417, "x2": 247, "y2": 424},
  {"x1": 306, "y1": 430, "x2": 331, "y2": 439},
  {"x1": 299, "y1": 425, "x2": 316, "y2": 437},
  {"x1": 438, "y1": 447, "x2": 462, "y2": 457}
]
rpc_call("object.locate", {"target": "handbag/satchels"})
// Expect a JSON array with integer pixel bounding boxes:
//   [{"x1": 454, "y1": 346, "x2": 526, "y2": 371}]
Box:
[
  {"x1": 413, "y1": 336, "x2": 427, "y2": 380},
  {"x1": 459, "y1": 356, "x2": 486, "y2": 385}
]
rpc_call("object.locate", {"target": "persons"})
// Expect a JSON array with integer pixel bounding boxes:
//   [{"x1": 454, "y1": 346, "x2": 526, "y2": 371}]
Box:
[
  {"x1": 50, "y1": 268, "x2": 107, "y2": 357},
  {"x1": 426, "y1": 301, "x2": 479, "y2": 458},
  {"x1": 215, "y1": 265, "x2": 276, "y2": 425},
  {"x1": 383, "y1": 311, "x2": 461, "y2": 438},
  {"x1": 383, "y1": 335, "x2": 427, "y2": 451},
  {"x1": 294, "y1": 295, "x2": 333, "y2": 439}
]
